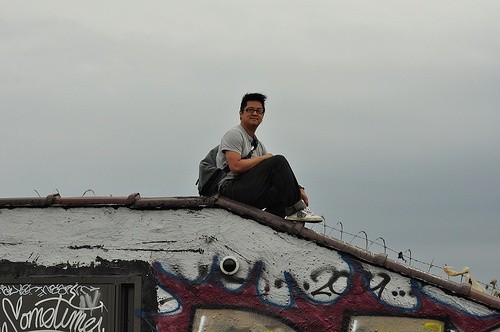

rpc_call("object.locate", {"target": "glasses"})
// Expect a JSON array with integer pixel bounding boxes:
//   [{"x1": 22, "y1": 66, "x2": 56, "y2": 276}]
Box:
[{"x1": 242, "y1": 107, "x2": 264, "y2": 114}]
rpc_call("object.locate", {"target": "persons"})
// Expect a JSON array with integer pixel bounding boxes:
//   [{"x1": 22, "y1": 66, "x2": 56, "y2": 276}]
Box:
[{"x1": 215, "y1": 92, "x2": 323, "y2": 222}]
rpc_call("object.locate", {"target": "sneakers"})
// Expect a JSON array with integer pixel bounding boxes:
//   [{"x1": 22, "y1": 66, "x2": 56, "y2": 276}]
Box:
[
  {"x1": 293, "y1": 221, "x2": 304, "y2": 228},
  {"x1": 284, "y1": 207, "x2": 323, "y2": 222}
]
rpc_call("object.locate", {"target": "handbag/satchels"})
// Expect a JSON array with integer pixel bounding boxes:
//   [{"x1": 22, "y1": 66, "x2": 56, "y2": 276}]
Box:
[{"x1": 195, "y1": 135, "x2": 258, "y2": 197}]
